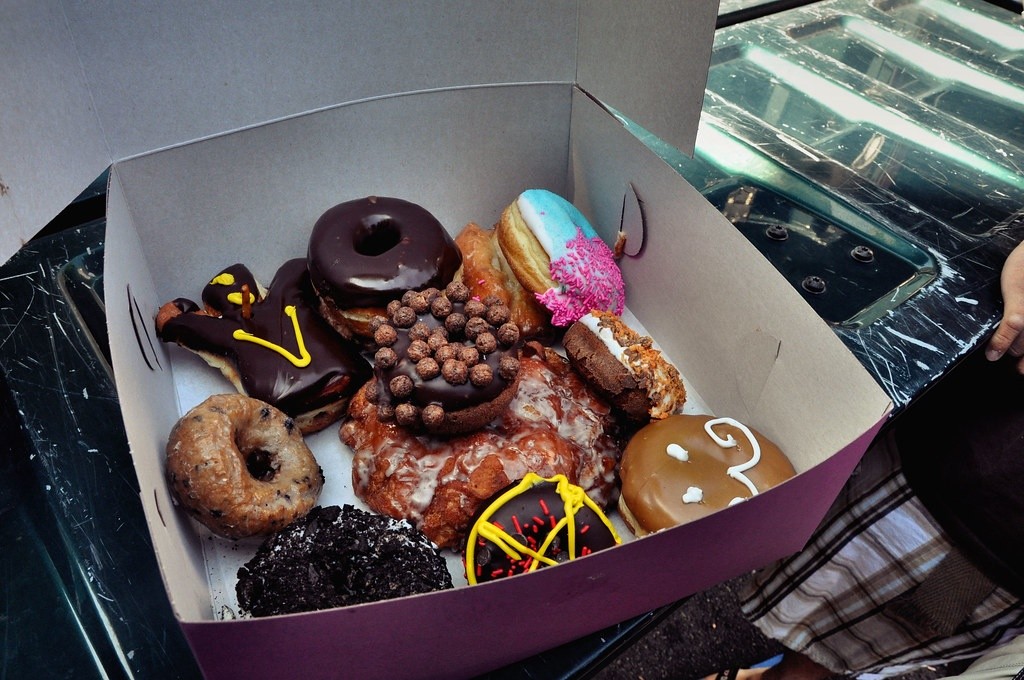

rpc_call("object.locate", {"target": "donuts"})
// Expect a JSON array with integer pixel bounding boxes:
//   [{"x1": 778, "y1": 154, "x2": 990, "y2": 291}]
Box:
[
  {"x1": 369, "y1": 282, "x2": 523, "y2": 434},
  {"x1": 497, "y1": 188, "x2": 624, "y2": 327},
  {"x1": 463, "y1": 473, "x2": 622, "y2": 586},
  {"x1": 164, "y1": 392, "x2": 323, "y2": 540},
  {"x1": 306, "y1": 196, "x2": 463, "y2": 338}
]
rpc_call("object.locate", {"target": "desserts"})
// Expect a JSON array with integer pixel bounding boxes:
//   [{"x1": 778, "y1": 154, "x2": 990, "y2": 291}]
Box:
[
  {"x1": 156, "y1": 224, "x2": 799, "y2": 619},
  {"x1": 562, "y1": 309, "x2": 686, "y2": 425}
]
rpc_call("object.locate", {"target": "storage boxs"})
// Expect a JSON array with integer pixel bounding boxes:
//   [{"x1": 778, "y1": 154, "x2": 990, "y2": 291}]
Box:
[{"x1": 0, "y1": 0, "x2": 895, "y2": 680}]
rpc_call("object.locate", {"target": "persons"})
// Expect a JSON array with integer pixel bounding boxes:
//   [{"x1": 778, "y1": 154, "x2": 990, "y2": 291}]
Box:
[{"x1": 697, "y1": 234, "x2": 1024, "y2": 679}]
